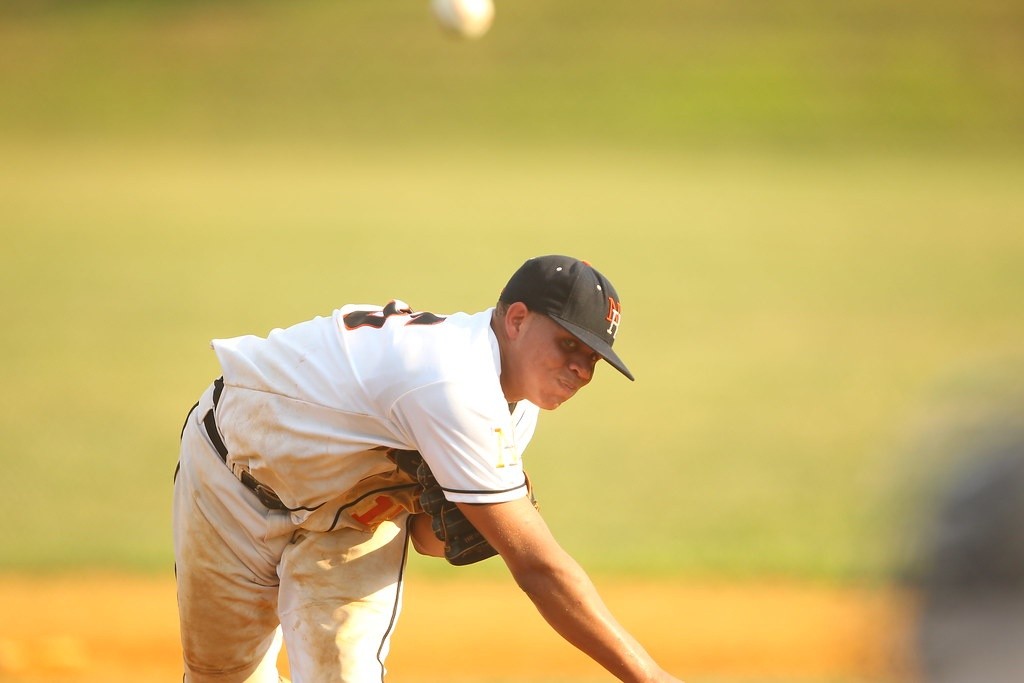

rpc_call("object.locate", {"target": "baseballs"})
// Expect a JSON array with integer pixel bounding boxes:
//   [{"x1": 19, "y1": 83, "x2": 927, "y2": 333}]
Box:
[{"x1": 429, "y1": 1, "x2": 494, "y2": 40}]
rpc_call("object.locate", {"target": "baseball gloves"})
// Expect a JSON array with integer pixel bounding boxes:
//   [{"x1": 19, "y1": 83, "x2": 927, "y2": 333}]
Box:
[{"x1": 385, "y1": 448, "x2": 538, "y2": 566}]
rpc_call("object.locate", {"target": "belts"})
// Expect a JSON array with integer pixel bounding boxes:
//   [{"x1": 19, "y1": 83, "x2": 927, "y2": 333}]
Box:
[{"x1": 201, "y1": 407, "x2": 282, "y2": 511}]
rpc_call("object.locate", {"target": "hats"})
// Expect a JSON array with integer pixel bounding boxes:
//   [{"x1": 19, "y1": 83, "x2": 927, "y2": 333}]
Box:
[{"x1": 505, "y1": 254, "x2": 635, "y2": 384}]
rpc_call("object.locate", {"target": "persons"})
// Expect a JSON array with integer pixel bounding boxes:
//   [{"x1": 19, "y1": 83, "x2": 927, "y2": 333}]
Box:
[{"x1": 173, "y1": 210, "x2": 697, "y2": 683}]
